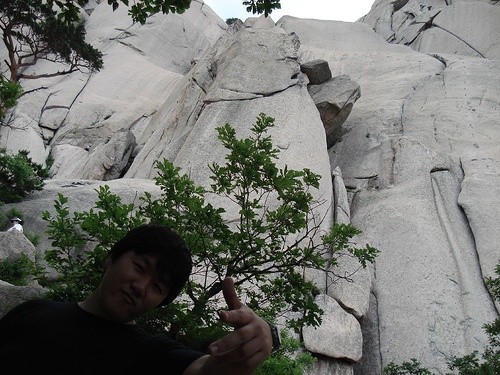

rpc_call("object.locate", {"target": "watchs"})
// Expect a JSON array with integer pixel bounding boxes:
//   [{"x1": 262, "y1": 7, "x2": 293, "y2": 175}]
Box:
[{"x1": 265, "y1": 319, "x2": 281, "y2": 352}]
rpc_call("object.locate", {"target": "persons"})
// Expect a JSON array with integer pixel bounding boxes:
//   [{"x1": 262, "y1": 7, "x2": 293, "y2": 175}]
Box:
[
  {"x1": 7, "y1": 217, "x2": 24, "y2": 233},
  {"x1": 0, "y1": 224, "x2": 272, "y2": 375}
]
